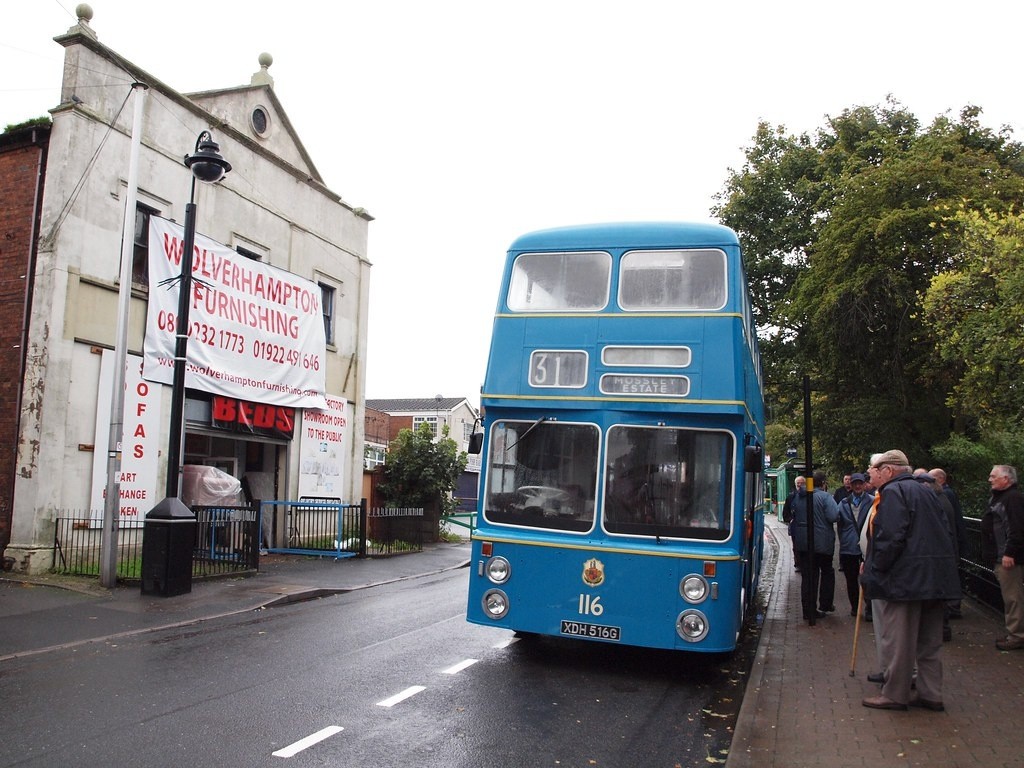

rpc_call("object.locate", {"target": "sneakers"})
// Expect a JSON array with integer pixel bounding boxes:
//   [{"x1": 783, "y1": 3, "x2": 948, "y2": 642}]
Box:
[{"x1": 996, "y1": 635, "x2": 1024, "y2": 650}]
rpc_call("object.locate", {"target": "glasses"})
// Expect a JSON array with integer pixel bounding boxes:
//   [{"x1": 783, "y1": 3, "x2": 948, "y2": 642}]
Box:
[
  {"x1": 876, "y1": 466, "x2": 888, "y2": 474},
  {"x1": 868, "y1": 465, "x2": 873, "y2": 471}
]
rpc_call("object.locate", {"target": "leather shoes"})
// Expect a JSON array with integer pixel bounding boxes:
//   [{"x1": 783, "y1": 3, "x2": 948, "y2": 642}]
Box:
[
  {"x1": 862, "y1": 695, "x2": 907, "y2": 711},
  {"x1": 908, "y1": 695, "x2": 945, "y2": 711}
]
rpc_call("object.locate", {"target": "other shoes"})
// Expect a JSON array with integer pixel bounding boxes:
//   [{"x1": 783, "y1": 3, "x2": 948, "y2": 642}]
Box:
[
  {"x1": 867, "y1": 672, "x2": 884, "y2": 682},
  {"x1": 865, "y1": 615, "x2": 872, "y2": 622},
  {"x1": 851, "y1": 609, "x2": 857, "y2": 616},
  {"x1": 803, "y1": 610, "x2": 825, "y2": 618},
  {"x1": 943, "y1": 625, "x2": 951, "y2": 641},
  {"x1": 839, "y1": 567, "x2": 844, "y2": 571},
  {"x1": 948, "y1": 613, "x2": 961, "y2": 619},
  {"x1": 795, "y1": 568, "x2": 800, "y2": 572},
  {"x1": 819, "y1": 605, "x2": 834, "y2": 612}
]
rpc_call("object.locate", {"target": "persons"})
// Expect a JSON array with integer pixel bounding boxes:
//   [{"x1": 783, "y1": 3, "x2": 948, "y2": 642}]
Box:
[
  {"x1": 783, "y1": 450, "x2": 959, "y2": 711},
  {"x1": 988, "y1": 465, "x2": 1024, "y2": 651}
]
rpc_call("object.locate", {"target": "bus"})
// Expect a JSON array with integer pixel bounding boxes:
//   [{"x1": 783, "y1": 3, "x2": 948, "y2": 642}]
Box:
[{"x1": 465, "y1": 219, "x2": 773, "y2": 658}]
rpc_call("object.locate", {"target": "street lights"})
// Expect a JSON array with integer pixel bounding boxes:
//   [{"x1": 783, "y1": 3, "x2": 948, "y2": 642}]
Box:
[{"x1": 139, "y1": 130, "x2": 234, "y2": 597}]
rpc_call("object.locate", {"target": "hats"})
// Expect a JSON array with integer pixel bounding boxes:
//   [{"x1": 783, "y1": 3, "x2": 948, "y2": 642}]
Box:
[
  {"x1": 915, "y1": 474, "x2": 935, "y2": 483},
  {"x1": 850, "y1": 473, "x2": 865, "y2": 483},
  {"x1": 873, "y1": 450, "x2": 909, "y2": 468},
  {"x1": 864, "y1": 473, "x2": 870, "y2": 480}
]
